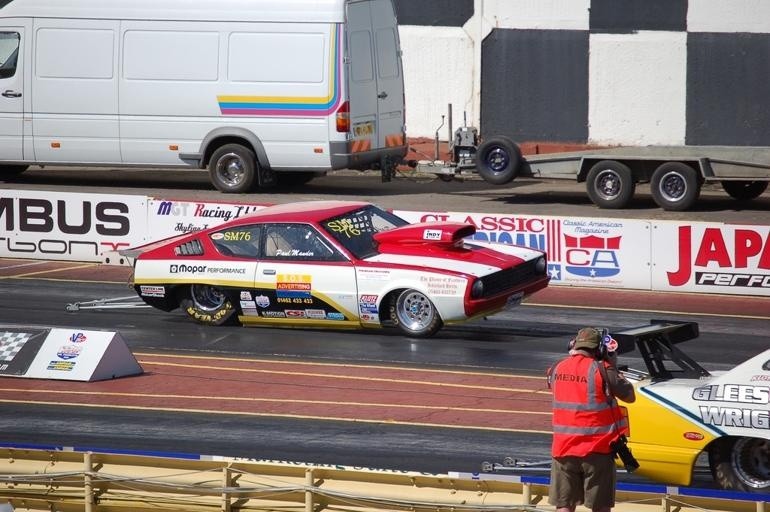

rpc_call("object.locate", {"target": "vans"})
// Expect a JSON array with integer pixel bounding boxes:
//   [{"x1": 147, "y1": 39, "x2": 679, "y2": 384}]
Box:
[{"x1": 0, "y1": 1, "x2": 410, "y2": 193}]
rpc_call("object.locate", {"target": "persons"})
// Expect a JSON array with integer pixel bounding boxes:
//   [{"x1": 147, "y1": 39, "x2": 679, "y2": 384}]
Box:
[{"x1": 547, "y1": 327, "x2": 636, "y2": 512}]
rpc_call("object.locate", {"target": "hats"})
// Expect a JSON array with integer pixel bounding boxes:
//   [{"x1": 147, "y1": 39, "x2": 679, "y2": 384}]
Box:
[{"x1": 573, "y1": 326, "x2": 602, "y2": 352}]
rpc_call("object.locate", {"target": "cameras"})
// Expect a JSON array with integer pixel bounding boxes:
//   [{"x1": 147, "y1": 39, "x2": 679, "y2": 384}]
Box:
[{"x1": 609, "y1": 434, "x2": 640, "y2": 473}]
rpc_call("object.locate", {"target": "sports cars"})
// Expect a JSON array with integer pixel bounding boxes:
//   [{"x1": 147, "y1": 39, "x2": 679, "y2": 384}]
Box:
[
  {"x1": 608, "y1": 319, "x2": 770, "y2": 495},
  {"x1": 118, "y1": 202, "x2": 550, "y2": 338}
]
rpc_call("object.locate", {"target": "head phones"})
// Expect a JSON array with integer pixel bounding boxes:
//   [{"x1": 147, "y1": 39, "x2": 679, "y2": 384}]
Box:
[{"x1": 568, "y1": 328, "x2": 607, "y2": 360}]
